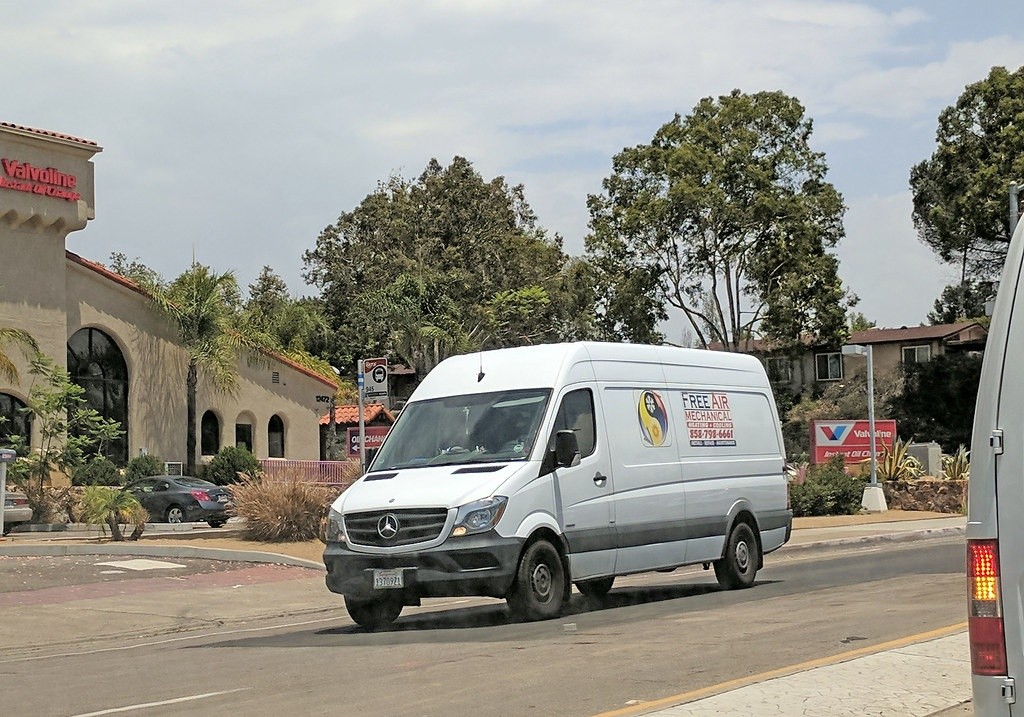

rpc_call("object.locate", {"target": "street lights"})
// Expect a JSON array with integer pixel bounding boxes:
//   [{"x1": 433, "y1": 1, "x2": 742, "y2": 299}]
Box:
[{"x1": 841, "y1": 344, "x2": 877, "y2": 486}]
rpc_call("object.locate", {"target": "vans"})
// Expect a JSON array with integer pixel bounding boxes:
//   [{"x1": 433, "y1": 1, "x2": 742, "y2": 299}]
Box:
[{"x1": 322, "y1": 339, "x2": 793, "y2": 629}]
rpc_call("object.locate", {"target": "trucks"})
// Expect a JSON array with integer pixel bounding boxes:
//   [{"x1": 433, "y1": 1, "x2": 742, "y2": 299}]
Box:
[{"x1": 966, "y1": 213, "x2": 1024, "y2": 717}]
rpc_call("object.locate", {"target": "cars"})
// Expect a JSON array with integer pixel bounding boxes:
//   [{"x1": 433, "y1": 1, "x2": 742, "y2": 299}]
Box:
[
  {"x1": 2, "y1": 491, "x2": 33, "y2": 537},
  {"x1": 105, "y1": 475, "x2": 235, "y2": 528}
]
